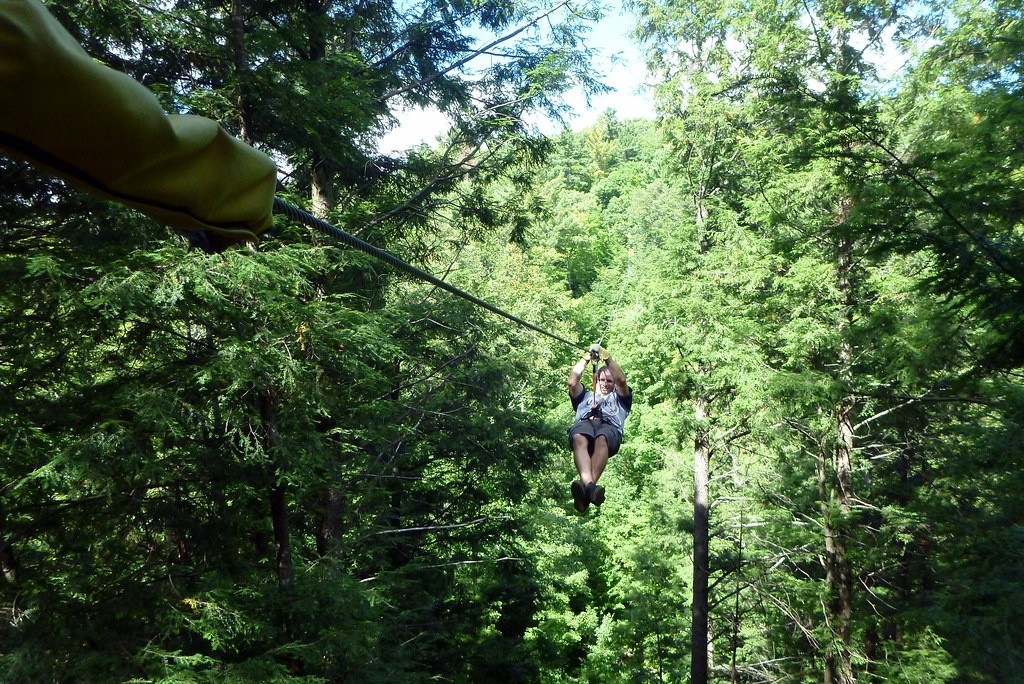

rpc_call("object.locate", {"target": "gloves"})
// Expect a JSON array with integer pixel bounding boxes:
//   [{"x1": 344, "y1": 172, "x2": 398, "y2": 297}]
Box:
[
  {"x1": 590, "y1": 344, "x2": 612, "y2": 362},
  {"x1": 582, "y1": 344, "x2": 595, "y2": 364}
]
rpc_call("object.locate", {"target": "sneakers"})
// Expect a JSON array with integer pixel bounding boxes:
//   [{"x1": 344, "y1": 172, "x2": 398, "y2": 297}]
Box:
[
  {"x1": 571, "y1": 480, "x2": 589, "y2": 512},
  {"x1": 590, "y1": 485, "x2": 606, "y2": 506}
]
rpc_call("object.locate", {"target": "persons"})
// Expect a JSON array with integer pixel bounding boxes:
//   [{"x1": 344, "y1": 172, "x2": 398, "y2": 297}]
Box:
[{"x1": 568, "y1": 342, "x2": 633, "y2": 513}]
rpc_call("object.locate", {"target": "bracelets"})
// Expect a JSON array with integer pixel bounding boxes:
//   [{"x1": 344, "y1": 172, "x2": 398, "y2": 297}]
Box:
[{"x1": 581, "y1": 355, "x2": 590, "y2": 364}]
